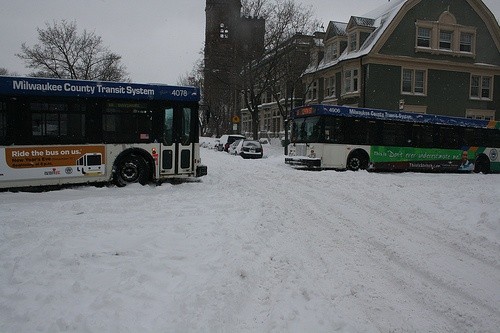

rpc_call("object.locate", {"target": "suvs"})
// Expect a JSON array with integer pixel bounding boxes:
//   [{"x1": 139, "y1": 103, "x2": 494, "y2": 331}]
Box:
[{"x1": 219, "y1": 135, "x2": 246, "y2": 151}]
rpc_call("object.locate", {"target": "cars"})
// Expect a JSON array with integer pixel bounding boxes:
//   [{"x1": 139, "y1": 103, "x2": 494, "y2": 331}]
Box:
[
  {"x1": 227, "y1": 139, "x2": 243, "y2": 155},
  {"x1": 235, "y1": 140, "x2": 263, "y2": 159}
]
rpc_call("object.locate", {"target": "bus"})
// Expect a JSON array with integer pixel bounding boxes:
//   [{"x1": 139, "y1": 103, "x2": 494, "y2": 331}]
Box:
[
  {"x1": 0, "y1": 75, "x2": 208, "y2": 189},
  {"x1": 285, "y1": 103, "x2": 500, "y2": 174}
]
